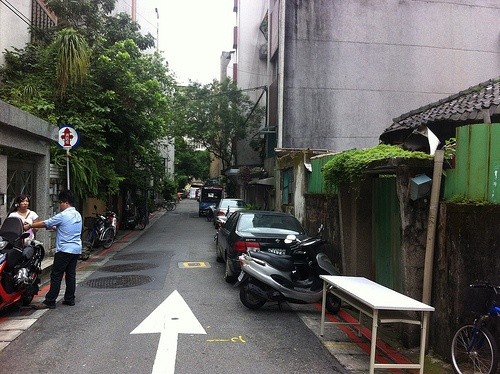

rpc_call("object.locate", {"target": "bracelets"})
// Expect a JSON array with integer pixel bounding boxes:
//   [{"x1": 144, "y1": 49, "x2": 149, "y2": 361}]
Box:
[{"x1": 29, "y1": 223, "x2": 32, "y2": 228}]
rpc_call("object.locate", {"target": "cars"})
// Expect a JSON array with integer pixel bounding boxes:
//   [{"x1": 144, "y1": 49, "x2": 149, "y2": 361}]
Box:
[
  {"x1": 215, "y1": 208, "x2": 307, "y2": 284},
  {"x1": 213, "y1": 198, "x2": 249, "y2": 230}
]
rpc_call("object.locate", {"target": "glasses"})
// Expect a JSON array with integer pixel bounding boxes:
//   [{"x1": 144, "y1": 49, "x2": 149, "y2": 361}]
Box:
[{"x1": 59, "y1": 201, "x2": 65, "y2": 204}]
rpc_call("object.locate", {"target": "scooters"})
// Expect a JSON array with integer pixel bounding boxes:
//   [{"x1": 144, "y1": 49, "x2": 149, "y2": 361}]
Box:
[
  {"x1": 0, "y1": 217, "x2": 45, "y2": 311},
  {"x1": 234, "y1": 224, "x2": 342, "y2": 315},
  {"x1": 120, "y1": 210, "x2": 147, "y2": 231},
  {"x1": 91, "y1": 204, "x2": 120, "y2": 237}
]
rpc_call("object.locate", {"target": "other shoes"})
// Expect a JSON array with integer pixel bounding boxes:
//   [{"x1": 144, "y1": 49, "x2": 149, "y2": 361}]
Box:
[
  {"x1": 32, "y1": 302, "x2": 55, "y2": 310},
  {"x1": 61, "y1": 301, "x2": 75, "y2": 306}
]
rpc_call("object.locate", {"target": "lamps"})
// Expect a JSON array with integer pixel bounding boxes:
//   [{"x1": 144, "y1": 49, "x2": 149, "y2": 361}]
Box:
[{"x1": 408, "y1": 174, "x2": 432, "y2": 201}]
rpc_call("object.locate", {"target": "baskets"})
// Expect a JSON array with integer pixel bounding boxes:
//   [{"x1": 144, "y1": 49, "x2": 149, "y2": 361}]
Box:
[{"x1": 84, "y1": 217, "x2": 96, "y2": 228}]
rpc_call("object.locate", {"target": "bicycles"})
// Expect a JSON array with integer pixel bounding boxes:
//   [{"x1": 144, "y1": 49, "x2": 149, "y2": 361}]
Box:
[
  {"x1": 154, "y1": 199, "x2": 176, "y2": 211},
  {"x1": 450, "y1": 279, "x2": 500, "y2": 374},
  {"x1": 205, "y1": 201, "x2": 215, "y2": 222},
  {"x1": 81, "y1": 212, "x2": 115, "y2": 252}
]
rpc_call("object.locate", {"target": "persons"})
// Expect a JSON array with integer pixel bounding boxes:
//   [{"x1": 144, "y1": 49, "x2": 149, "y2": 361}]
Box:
[
  {"x1": 8, "y1": 193, "x2": 39, "y2": 241},
  {"x1": 177, "y1": 192, "x2": 181, "y2": 204},
  {"x1": 24, "y1": 190, "x2": 82, "y2": 309}
]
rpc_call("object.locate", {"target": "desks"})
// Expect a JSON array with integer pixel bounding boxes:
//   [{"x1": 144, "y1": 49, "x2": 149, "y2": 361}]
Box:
[{"x1": 319, "y1": 275, "x2": 435, "y2": 374}]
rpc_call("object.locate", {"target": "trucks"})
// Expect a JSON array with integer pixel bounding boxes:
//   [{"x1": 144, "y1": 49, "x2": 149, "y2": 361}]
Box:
[{"x1": 197, "y1": 186, "x2": 223, "y2": 217}]
